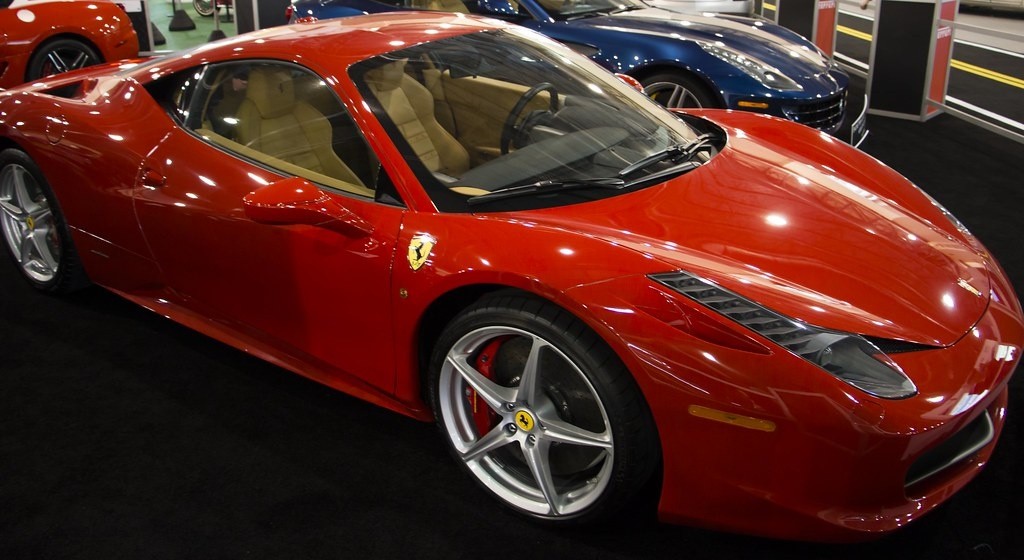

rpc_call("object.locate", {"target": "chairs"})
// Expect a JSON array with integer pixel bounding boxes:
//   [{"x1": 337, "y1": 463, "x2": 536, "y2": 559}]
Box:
[
  {"x1": 364, "y1": 61, "x2": 471, "y2": 179},
  {"x1": 235, "y1": 67, "x2": 366, "y2": 188},
  {"x1": 420, "y1": 0, "x2": 471, "y2": 14}
]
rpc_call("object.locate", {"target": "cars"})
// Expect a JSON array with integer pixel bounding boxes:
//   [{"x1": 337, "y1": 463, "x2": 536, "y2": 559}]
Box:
[{"x1": 0, "y1": 0, "x2": 140, "y2": 92}]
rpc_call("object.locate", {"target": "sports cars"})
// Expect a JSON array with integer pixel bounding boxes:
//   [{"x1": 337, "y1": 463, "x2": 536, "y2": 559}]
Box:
[
  {"x1": 1, "y1": 14, "x2": 1024, "y2": 546},
  {"x1": 282, "y1": 1, "x2": 853, "y2": 144}
]
